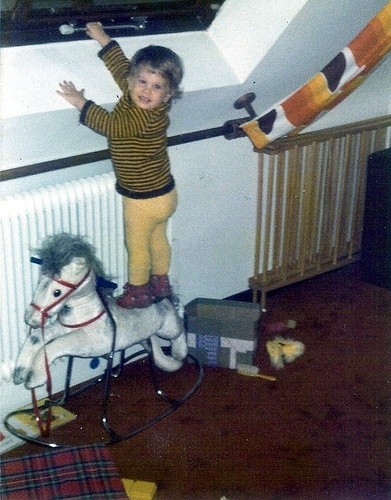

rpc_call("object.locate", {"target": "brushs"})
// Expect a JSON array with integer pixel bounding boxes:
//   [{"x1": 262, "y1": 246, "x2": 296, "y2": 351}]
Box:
[{"x1": 236, "y1": 363, "x2": 276, "y2": 381}]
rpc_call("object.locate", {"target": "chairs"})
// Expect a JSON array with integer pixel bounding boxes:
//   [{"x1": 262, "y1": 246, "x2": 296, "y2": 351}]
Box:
[{"x1": 0, "y1": 441, "x2": 129, "y2": 500}]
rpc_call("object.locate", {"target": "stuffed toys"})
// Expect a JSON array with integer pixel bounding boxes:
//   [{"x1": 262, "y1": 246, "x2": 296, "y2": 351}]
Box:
[{"x1": 11, "y1": 233, "x2": 189, "y2": 390}]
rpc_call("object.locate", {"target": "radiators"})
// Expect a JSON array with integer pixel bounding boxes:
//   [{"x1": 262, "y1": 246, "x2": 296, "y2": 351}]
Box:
[{"x1": 0, "y1": 171, "x2": 173, "y2": 385}]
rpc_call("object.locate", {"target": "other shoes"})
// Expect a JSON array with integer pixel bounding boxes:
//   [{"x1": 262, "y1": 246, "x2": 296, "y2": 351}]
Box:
[
  {"x1": 116, "y1": 282, "x2": 151, "y2": 309},
  {"x1": 149, "y1": 275, "x2": 172, "y2": 299}
]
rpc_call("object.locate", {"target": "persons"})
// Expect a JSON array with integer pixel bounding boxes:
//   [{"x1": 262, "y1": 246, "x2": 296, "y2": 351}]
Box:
[{"x1": 55, "y1": 22, "x2": 184, "y2": 308}]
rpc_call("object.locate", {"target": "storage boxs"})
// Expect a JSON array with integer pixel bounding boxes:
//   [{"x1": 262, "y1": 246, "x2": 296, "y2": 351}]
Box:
[{"x1": 183, "y1": 297, "x2": 261, "y2": 371}]
